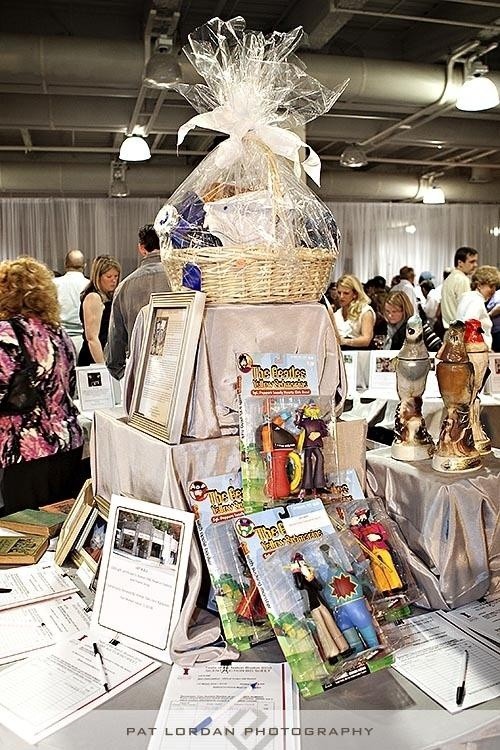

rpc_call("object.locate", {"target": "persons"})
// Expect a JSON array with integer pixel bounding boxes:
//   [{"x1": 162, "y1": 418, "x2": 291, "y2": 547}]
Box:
[
  {"x1": 0, "y1": 223, "x2": 173, "y2": 516},
  {"x1": 325, "y1": 246, "x2": 500, "y2": 353}
]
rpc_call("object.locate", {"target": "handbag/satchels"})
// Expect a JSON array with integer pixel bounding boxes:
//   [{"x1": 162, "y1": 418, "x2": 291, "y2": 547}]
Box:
[{"x1": 0, "y1": 361, "x2": 41, "y2": 413}]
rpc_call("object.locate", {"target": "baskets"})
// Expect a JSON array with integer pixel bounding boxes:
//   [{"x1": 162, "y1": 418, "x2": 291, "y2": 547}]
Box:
[{"x1": 159, "y1": 134, "x2": 338, "y2": 306}]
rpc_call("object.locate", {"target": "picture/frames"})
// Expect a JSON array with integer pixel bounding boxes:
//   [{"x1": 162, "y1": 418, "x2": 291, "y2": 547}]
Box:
[{"x1": 124, "y1": 290, "x2": 206, "y2": 445}]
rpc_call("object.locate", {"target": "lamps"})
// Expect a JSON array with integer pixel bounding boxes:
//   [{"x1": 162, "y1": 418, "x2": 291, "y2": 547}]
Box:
[
  {"x1": 110, "y1": 11, "x2": 182, "y2": 198},
  {"x1": 315, "y1": 39, "x2": 500, "y2": 205}
]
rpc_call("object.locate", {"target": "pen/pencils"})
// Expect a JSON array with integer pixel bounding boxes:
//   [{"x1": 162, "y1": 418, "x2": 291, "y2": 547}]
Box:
[
  {"x1": 93, "y1": 643, "x2": 108, "y2": 693},
  {"x1": 457, "y1": 650, "x2": 467, "y2": 704},
  {"x1": 196, "y1": 682, "x2": 257, "y2": 732}
]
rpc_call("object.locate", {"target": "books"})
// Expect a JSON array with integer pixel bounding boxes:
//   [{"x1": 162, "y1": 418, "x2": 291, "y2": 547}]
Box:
[{"x1": 182, "y1": 351, "x2": 426, "y2": 700}]
